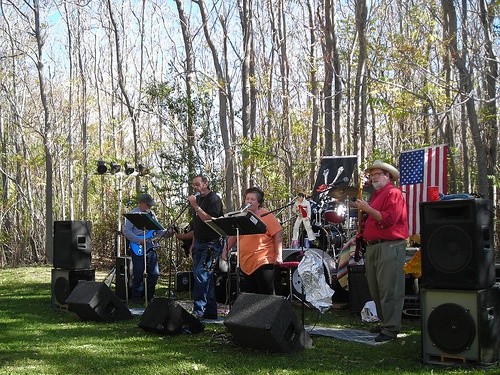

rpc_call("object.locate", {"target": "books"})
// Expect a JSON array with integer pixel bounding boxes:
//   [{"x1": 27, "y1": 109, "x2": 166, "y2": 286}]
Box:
[
  {"x1": 205, "y1": 210, "x2": 267, "y2": 237},
  {"x1": 123, "y1": 212, "x2": 164, "y2": 230}
]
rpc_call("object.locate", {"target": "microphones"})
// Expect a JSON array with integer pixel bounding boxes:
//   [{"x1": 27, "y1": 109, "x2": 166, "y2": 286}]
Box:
[
  {"x1": 241, "y1": 203, "x2": 252, "y2": 212},
  {"x1": 332, "y1": 177, "x2": 349, "y2": 186},
  {"x1": 195, "y1": 192, "x2": 201, "y2": 197}
]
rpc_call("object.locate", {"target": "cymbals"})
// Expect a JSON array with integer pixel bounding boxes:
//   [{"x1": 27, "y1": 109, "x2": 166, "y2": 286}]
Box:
[{"x1": 328, "y1": 186, "x2": 357, "y2": 199}]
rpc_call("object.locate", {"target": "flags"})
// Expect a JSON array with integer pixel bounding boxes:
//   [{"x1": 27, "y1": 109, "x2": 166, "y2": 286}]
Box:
[
  {"x1": 311, "y1": 155, "x2": 357, "y2": 225},
  {"x1": 399, "y1": 144, "x2": 448, "y2": 235}
]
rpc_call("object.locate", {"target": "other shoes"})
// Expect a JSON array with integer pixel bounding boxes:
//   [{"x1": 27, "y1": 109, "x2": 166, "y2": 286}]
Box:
[
  {"x1": 375, "y1": 333, "x2": 397, "y2": 342},
  {"x1": 132, "y1": 297, "x2": 155, "y2": 304},
  {"x1": 370, "y1": 325, "x2": 381, "y2": 333},
  {"x1": 192, "y1": 308, "x2": 218, "y2": 320}
]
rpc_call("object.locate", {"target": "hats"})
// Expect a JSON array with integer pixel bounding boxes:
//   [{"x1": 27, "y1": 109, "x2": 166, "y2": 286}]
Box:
[
  {"x1": 139, "y1": 194, "x2": 155, "y2": 206},
  {"x1": 365, "y1": 160, "x2": 400, "y2": 181}
]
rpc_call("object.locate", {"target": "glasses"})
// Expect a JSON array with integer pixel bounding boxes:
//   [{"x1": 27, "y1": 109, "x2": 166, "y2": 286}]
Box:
[{"x1": 370, "y1": 173, "x2": 384, "y2": 177}]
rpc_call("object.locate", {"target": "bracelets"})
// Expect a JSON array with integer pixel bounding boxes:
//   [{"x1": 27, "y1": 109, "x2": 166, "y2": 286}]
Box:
[{"x1": 196, "y1": 207, "x2": 200, "y2": 212}]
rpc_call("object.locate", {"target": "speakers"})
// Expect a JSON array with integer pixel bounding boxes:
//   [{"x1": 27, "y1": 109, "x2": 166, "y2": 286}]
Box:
[
  {"x1": 138, "y1": 297, "x2": 206, "y2": 337},
  {"x1": 53, "y1": 220, "x2": 92, "y2": 269},
  {"x1": 50, "y1": 268, "x2": 95, "y2": 312},
  {"x1": 347, "y1": 264, "x2": 369, "y2": 313},
  {"x1": 224, "y1": 292, "x2": 304, "y2": 356},
  {"x1": 66, "y1": 280, "x2": 134, "y2": 322},
  {"x1": 421, "y1": 283, "x2": 500, "y2": 367},
  {"x1": 175, "y1": 271, "x2": 194, "y2": 292},
  {"x1": 115, "y1": 256, "x2": 133, "y2": 300},
  {"x1": 419, "y1": 198, "x2": 496, "y2": 292}
]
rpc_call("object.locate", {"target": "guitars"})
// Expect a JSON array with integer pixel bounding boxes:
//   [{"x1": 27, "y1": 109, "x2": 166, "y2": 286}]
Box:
[
  {"x1": 353, "y1": 171, "x2": 365, "y2": 263},
  {"x1": 172, "y1": 225, "x2": 192, "y2": 257},
  {"x1": 129, "y1": 222, "x2": 190, "y2": 257}
]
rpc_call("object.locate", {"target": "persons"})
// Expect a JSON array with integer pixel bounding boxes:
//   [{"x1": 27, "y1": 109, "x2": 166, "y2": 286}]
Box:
[
  {"x1": 188, "y1": 175, "x2": 224, "y2": 321},
  {"x1": 355, "y1": 160, "x2": 408, "y2": 342},
  {"x1": 292, "y1": 193, "x2": 316, "y2": 241},
  {"x1": 176, "y1": 220, "x2": 193, "y2": 258},
  {"x1": 222, "y1": 187, "x2": 283, "y2": 295},
  {"x1": 123, "y1": 194, "x2": 174, "y2": 302}
]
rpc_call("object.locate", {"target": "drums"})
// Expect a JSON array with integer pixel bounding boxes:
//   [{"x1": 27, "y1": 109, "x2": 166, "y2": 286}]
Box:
[
  {"x1": 324, "y1": 201, "x2": 347, "y2": 223},
  {"x1": 299, "y1": 225, "x2": 342, "y2": 253}
]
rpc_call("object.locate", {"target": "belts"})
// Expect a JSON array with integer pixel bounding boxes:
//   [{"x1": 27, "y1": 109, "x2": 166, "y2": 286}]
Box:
[{"x1": 365, "y1": 238, "x2": 403, "y2": 246}]
